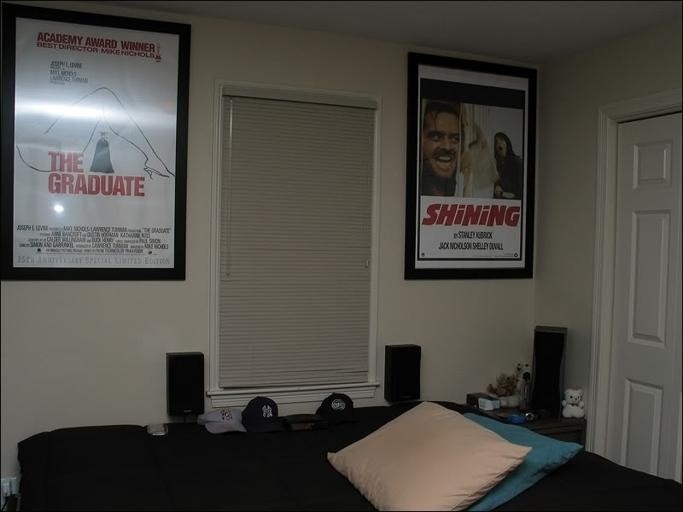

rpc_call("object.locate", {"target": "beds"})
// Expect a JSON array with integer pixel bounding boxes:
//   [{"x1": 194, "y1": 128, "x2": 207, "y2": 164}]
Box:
[{"x1": 18, "y1": 404, "x2": 655, "y2": 511}]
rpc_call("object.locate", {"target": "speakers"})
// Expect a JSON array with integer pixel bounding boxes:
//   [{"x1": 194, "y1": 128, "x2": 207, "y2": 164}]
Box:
[
  {"x1": 241, "y1": 396, "x2": 278, "y2": 425},
  {"x1": 384, "y1": 344, "x2": 421, "y2": 402},
  {"x1": 166, "y1": 352, "x2": 205, "y2": 416},
  {"x1": 315, "y1": 393, "x2": 353, "y2": 415},
  {"x1": 530, "y1": 326, "x2": 568, "y2": 422}
]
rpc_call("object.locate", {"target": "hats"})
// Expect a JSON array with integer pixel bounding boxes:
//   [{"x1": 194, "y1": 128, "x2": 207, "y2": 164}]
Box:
[
  {"x1": 243, "y1": 398, "x2": 283, "y2": 432},
  {"x1": 315, "y1": 393, "x2": 356, "y2": 425},
  {"x1": 197, "y1": 409, "x2": 247, "y2": 434}
]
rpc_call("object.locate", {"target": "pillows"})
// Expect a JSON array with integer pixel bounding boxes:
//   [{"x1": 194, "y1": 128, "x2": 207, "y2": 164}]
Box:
[{"x1": 327, "y1": 401, "x2": 583, "y2": 511}]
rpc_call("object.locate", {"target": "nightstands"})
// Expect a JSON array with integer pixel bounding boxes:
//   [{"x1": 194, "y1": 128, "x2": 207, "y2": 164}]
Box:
[{"x1": 484, "y1": 408, "x2": 587, "y2": 451}]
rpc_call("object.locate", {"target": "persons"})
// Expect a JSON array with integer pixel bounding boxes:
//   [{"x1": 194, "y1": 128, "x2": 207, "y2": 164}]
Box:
[
  {"x1": 420, "y1": 97, "x2": 473, "y2": 197},
  {"x1": 492, "y1": 130, "x2": 522, "y2": 199}
]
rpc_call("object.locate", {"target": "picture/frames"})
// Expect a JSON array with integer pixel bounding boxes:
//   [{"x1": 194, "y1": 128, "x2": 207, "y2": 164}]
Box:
[
  {"x1": 404, "y1": 51, "x2": 538, "y2": 279},
  {"x1": 1, "y1": 2, "x2": 191, "y2": 281}
]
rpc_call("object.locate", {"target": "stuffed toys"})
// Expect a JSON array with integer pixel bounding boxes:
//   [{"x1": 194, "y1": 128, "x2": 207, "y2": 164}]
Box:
[
  {"x1": 486, "y1": 361, "x2": 530, "y2": 409},
  {"x1": 560, "y1": 387, "x2": 585, "y2": 420}
]
